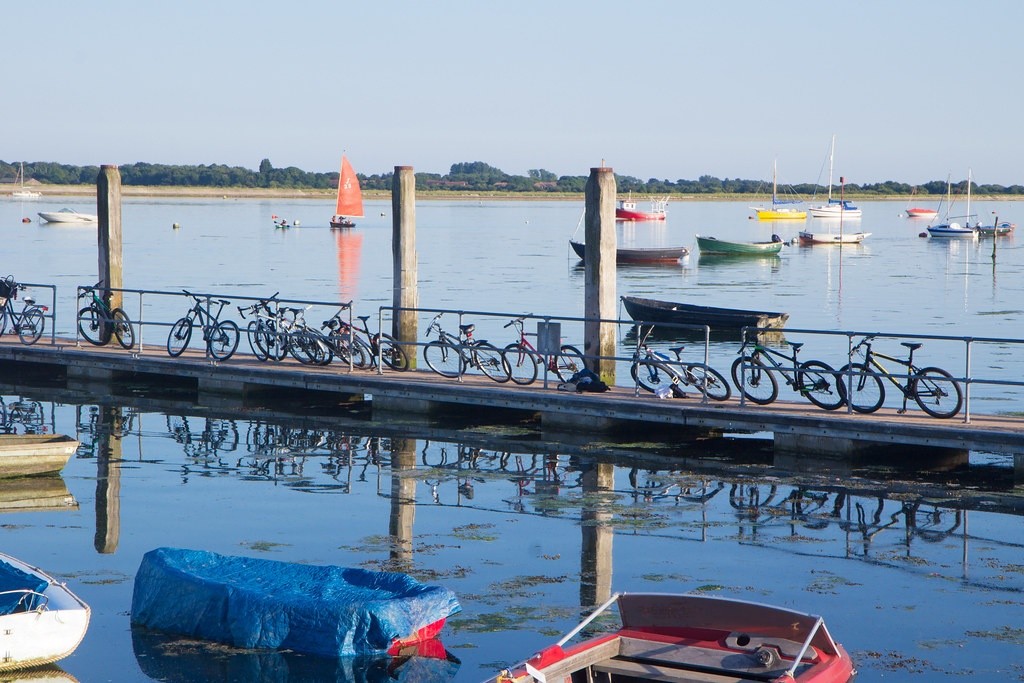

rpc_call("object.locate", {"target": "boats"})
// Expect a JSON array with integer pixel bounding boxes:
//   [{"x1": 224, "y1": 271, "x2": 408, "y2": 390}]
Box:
[
  {"x1": 275, "y1": 225, "x2": 290, "y2": 228},
  {"x1": 12, "y1": 190, "x2": 42, "y2": 202},
  {"x1": 619, "y1": 295, "x2": 790, "y2": 340},
  {"x1": 0, "y1": 552, "x2": 92, "y2": 675},
  {"x1": 615, "y1": 189, "x2": 671, "y2": 222},
  {"x1": 0, "y1": 433, "x2": 80, "y2": 477},
  {"x1": 696, "y1": 235, "x2": 783, "y2": 257},
  {"x1": 131, "y1": 548, "x2": 464, "y2": 653},
  {"x1": 0, "y1": 475, "x2": 81, "y2": 512},
  {"x1": 569, "y1": 238, "x2": 689, "y2": 264},
  {"x1": 38, "y1": 206, "x2": 98, "y2": 225},
  {"x1": 485, "y1": 590, "x2": 857, "y2": 683},
  {"x1": 798, "y1": 230, "x2": 872, "y2": 243}
]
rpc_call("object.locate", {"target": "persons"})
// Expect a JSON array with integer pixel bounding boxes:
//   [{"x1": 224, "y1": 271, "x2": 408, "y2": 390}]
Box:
[
  {"x1": 332, "y1": 216, "x2": 352, "y2": 224},
  {"x1": 282, "y1": 219, "x2": 287, "y2": 226}
]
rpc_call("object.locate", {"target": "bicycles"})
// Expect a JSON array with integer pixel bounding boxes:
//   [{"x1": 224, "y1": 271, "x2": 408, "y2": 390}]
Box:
[
  {"x1": 0, "y1": 277, "x2": 967, "y2": 421},
  {"x1": 0, "y1": 397, "x2": 963, "y2": 560}
]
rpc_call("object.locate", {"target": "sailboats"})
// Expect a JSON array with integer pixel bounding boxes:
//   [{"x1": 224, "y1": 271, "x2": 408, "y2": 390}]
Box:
[
  {"x1": 748, "y1": 156, "x2": 807, "y2": 219},
  {"x1": 905, "y1": 173, "x2": 1016, "y2": 238},
  {"x1": 329, "y1": 149, "x2": 365, "y2": 228},
  {"x1": 809, "y1": 134, "x2": 862, "y2": 218}
]
